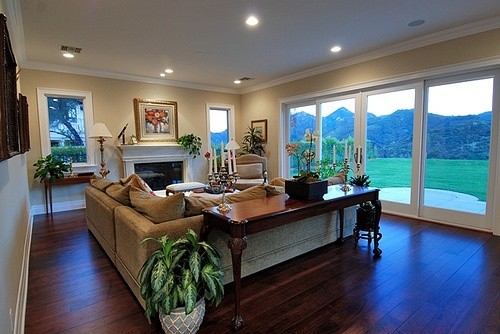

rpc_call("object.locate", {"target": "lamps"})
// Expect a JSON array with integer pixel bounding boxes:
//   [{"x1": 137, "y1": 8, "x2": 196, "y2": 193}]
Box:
[
  {"x1": 88, "y1": 123, "x2": 113, "y2": 178},
  {"x1": 233, "y1": 77, "x2": 250, "y2": 84},
  {"x1": 61, "y1": 46, "x2": 82, "y2": 58}
]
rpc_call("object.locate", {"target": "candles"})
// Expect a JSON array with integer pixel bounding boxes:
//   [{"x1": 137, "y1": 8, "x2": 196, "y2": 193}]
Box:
[
  {"x1": 332, "y1": 140, "x2": 363, "y2": 168},
  {"x1": 205, "y1": 140, "x2": 238, "y2": 174}
]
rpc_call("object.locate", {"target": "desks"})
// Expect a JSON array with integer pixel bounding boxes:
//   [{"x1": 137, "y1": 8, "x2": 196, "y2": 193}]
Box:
[
  {"x1": 45, "y1": 174, "x2": 96, "y2": 217},
  {"x1": 201, "y1": 180, "x2": 382, "y2": 331}
]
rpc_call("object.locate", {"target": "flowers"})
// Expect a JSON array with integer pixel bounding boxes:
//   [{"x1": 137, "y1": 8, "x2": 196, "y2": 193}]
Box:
[{"x1": 285, "y1": 127, "x2": 320, "y2": 176}]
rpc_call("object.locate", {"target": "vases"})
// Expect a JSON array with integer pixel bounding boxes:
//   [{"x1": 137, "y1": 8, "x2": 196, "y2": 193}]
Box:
[{"x1": 285, "y1": 180, "x2": 328, "y2": 200}]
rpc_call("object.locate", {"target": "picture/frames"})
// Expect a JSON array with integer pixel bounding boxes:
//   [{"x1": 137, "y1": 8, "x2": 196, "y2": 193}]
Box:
[{"x1": 251, "y1": 118, "x2": 267, "y2": 142}]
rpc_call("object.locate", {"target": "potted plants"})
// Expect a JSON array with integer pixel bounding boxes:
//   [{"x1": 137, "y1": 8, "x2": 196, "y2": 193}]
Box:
[
  {"x1": 33, "y1": 154, "x2": 73, "y2": 187},
  {"x1": 137, "y1": 227, "x2": 224, "y2": 334}
]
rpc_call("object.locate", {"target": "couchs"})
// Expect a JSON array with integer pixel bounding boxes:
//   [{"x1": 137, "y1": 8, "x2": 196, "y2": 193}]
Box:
[{"x1": 85, "y1": 154, "x2": 360, "y2": 315}]
